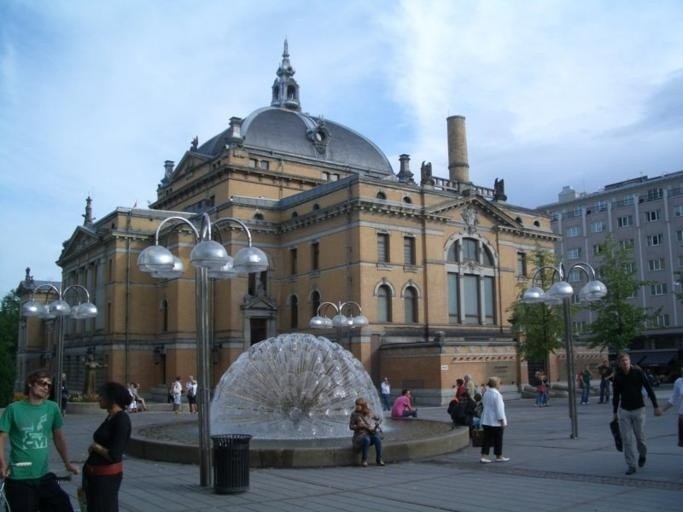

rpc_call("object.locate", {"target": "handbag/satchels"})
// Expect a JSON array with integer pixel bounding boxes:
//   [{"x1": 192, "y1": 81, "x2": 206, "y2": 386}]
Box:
[
  {"x1": 359, "y1": 415, "x2": 379, "y2": 431},
  {"x1": 537, "y1": 384, "x2": 545, "y2": 392},
  {"x1": 472, "y1": 429, "x2": 494, "y2": 448}
]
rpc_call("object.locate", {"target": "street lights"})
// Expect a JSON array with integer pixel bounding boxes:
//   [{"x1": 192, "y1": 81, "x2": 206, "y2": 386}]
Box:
[
  {"x1": 135, "y1": 210, "x2": 270, "y2": 488},
  {"x1": 310, "y1": 299, "x2": 369, "y2": 344},
  {"x1": 22, "y1": 283, "x2": 100, "y2": 412},
  {"x1": 522, "y1": 262, "x2": 607, "y2": 441}
]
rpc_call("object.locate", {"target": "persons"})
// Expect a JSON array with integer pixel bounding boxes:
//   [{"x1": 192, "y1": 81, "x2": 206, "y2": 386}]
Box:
[
  {"x1": 82, "y1": 382, "x2": 132, "y2": 512},
  {"x1": 448, "y1": 374, "x2": 510, "y2": 464},
  {"x1": 126, "y1": 380, "x2": 148, "y2": 414},
  {"x1": 533, "y1": 352, "x2": 682, "y2": 475},
  {"x1": 0, "y1": 368, "x2": 77, "y2": 512},
  {"x1": 350, "y1": 398, "x2": 385, "y2": 468},
  {"x1": 61, "y1": 373, "x2": 70, "y2": 418},
  {"x1": 380, "y1": 376, "x2": 391, "y2": 411},
  {"x1": 167, "y1": 375, "x2": 198, "y2": 415},
  {"x1": 391, "y1": 389, "x2": 418, "y2": 419}
]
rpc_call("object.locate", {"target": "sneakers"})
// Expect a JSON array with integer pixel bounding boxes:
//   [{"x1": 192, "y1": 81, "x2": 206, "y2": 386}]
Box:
[
  {"x1": 361, "y1": 461, "x2": 368, "y2": 467},
  {"x1": 480, "y1": 458, "x2": 492, "y2": 463},
  {"x1": 377, "y1": 460, "x2": 385, "y2": 465},
  {"x1": 639, "y1": 455, "x2": 646, "y2": 467},
  {"x1": 496, "y1": 457, "x2": 510, "y2": 462},
  {"x1": 625, "y1": 467, "x2": 635, "y2": 474}
]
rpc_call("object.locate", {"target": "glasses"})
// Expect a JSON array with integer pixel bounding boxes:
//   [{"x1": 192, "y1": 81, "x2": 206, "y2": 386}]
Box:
[{"x1": 36, "y1": 379, "x2": 52, "y2": 388}]
